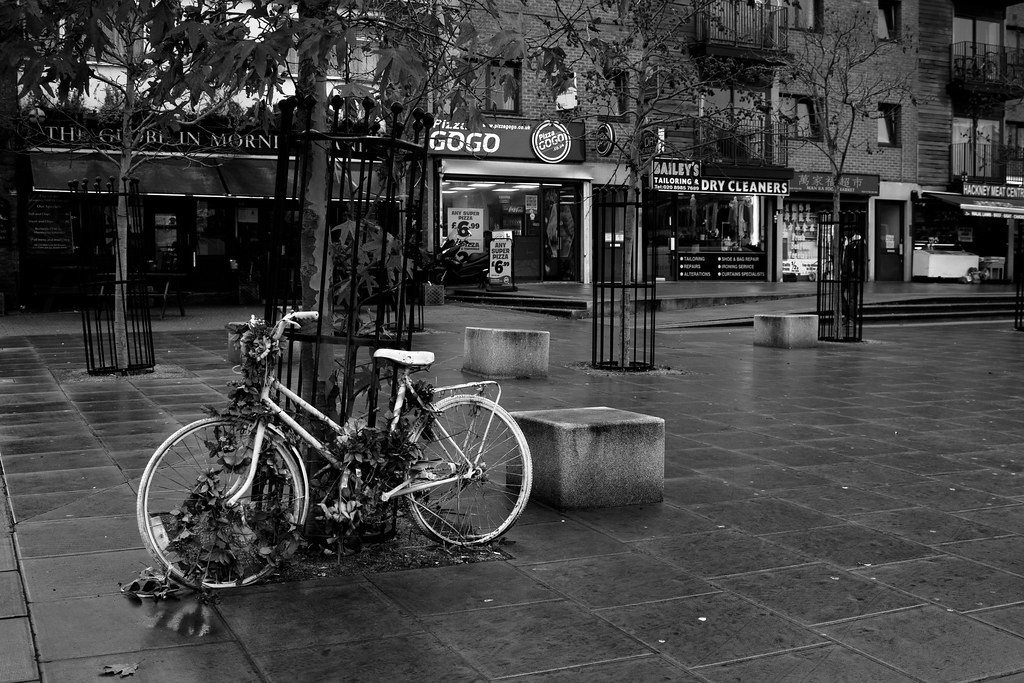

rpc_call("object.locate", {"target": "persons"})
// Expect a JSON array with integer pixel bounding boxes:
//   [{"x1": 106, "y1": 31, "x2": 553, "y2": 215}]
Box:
[
  {"x1": 839, "y1": 235, "x2": 857, "y2": 327},
  {"x1": 928, "y1": 237, "x2": 934, "y2": 242}
]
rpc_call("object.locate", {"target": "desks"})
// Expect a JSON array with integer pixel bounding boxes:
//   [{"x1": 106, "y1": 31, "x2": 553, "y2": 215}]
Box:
[{"x1": 92, "y1": 271, "x2": 186, "y2": 320}]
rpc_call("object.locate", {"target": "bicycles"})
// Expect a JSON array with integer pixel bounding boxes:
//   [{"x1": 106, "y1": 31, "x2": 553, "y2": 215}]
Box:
[{"x1": 136, "y1": 310, "x2": 534, "y2": 591}]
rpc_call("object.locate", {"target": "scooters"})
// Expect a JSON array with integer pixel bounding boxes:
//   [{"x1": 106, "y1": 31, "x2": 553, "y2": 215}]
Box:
[{"x1": 433, "y1": 230, "x2": 491, "y2": 288}]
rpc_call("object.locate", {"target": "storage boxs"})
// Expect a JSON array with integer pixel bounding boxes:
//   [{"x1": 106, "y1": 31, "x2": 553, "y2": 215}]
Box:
[{"x1": 421, "y1": 285, "x2": 444, "y2": 306}]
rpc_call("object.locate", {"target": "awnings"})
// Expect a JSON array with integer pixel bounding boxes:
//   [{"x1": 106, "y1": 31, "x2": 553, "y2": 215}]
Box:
[{"x1": 922, "y1": 192, "x2": 1024, "y2": 220}]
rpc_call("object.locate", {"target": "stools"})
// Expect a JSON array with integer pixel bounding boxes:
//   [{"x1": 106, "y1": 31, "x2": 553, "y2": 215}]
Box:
[{"x1": 983, "y1": 263, "x2": 1004, "y2": 279}]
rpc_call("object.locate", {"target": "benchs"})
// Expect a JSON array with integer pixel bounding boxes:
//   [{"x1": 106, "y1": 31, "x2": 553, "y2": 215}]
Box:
[
  {"x1": 753, "y1": 313, "x2": 819, "y2": 348},
  {"x1": 83, "y1": 290, "x2": 195, "y2": 299},
  {"x1": 506, "y1": 407, "x2": 667, "y2": 510},
  {"x1": 460, "y1": 327, "x2": 552, "y2": 379},
  {"x1": 226, "y1": 322, "x2": 300, "y2": 364}
]
